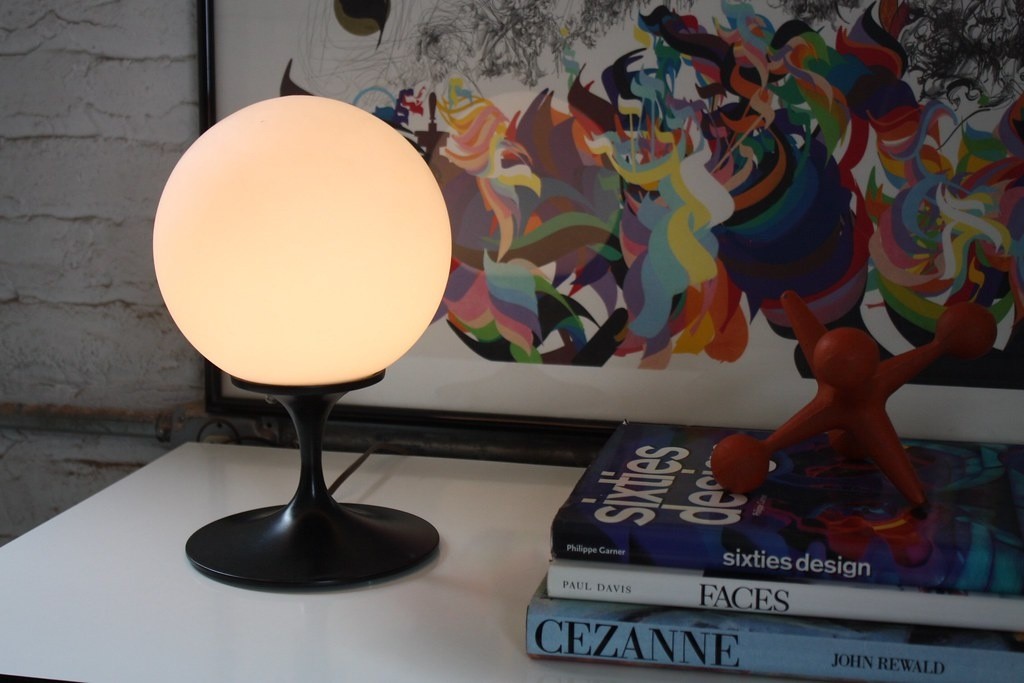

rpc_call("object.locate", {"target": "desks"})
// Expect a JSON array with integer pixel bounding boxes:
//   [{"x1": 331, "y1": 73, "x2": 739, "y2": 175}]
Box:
[{"x1": 1, "y1": 437, "x2": 814, "y2": 683}]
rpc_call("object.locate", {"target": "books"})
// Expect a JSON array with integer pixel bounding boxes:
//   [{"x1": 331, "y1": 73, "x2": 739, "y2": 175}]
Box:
[{"x1": 525, "y1": 422, "x2": 1024, "y2": 683}]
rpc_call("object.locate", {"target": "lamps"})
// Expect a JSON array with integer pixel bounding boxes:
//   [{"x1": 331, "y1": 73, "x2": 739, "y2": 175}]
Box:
[{"x1": 151, "y1": 94, "x2": 451, "y2": 594}]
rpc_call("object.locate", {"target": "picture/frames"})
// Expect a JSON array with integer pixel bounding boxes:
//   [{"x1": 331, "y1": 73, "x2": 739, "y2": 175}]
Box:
[{"x1": 193, "y1": 0, "x2": 1024, "y2": 445}]
